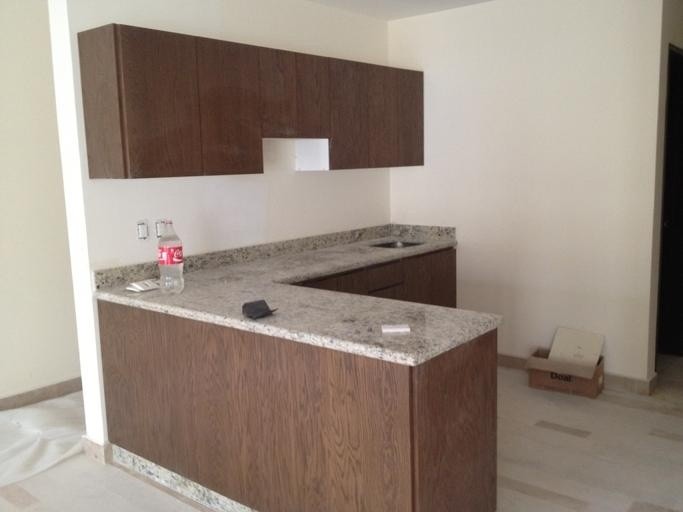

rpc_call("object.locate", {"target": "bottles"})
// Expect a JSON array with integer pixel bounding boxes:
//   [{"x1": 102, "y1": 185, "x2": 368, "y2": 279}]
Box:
[{"x1": 158, "y1": 218, "x2": 184, "y2": 295}]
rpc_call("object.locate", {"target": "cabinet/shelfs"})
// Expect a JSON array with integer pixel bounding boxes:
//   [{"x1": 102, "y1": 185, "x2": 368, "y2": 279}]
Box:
[
  {"x1": 76, "y1": 21, "x2": 423, "y2": 180},
  {"x1": 293, "y1": 246, "x2": 458, "y2": 308}
]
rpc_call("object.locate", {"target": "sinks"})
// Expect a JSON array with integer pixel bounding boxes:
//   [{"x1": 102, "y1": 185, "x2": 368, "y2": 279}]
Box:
[{"x1": 369, "y1": 239, "x2": 427, "y2": 249}]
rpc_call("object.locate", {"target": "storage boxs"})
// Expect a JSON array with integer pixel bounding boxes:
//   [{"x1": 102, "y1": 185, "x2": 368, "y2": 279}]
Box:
[{"x1": 524, "y1": 345, "x2": 605, "y2": 398}]
